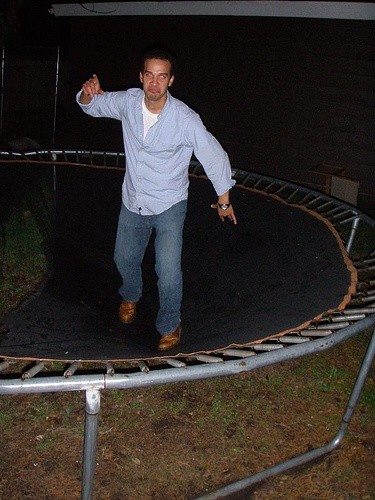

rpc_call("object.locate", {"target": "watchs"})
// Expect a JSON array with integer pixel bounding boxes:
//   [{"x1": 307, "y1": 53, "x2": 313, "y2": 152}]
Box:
[{"x1": 217, "y1": 201, "x2": 231, "y2": 211}]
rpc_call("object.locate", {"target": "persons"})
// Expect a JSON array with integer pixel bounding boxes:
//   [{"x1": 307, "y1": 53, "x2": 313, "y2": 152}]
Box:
[{"x1": 75, "y1": 49, "x2": 238, "y2": 351}]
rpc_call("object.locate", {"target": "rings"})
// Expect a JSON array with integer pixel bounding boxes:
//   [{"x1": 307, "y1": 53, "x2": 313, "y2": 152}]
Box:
[{"x1": 86, "y1": 81, "x2": 90, "y2": 85}]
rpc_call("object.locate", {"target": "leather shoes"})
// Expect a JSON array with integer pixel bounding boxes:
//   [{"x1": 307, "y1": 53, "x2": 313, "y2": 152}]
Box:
[
  {"x1": 119, "y1": 301, "x2": 137, "y2": 323},
  {"x1": 159, "y1": 326, "x2": 181, "y2": 351}
]
